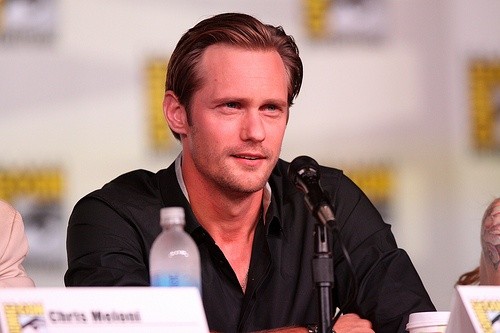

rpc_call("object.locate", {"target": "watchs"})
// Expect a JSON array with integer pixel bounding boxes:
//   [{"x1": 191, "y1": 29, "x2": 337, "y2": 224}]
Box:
[{"x1": 305, "y1": 319, "x2": 321, "y2": 333}]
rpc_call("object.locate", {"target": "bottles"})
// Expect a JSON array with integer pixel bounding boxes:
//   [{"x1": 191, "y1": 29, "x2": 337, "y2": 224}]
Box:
[{"x1": 149, "y1": 207, "x2": 202, "y2": 288}]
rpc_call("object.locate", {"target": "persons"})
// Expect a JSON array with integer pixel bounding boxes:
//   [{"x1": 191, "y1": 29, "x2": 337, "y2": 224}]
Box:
[{"x1": 65, "y1": 14, "x2": 436, "y2": 333}]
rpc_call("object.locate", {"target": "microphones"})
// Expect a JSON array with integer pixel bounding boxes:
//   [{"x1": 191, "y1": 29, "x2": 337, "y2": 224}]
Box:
[{"x1": 287, "y1": 156, "x2": 337, "y2": 233}]
